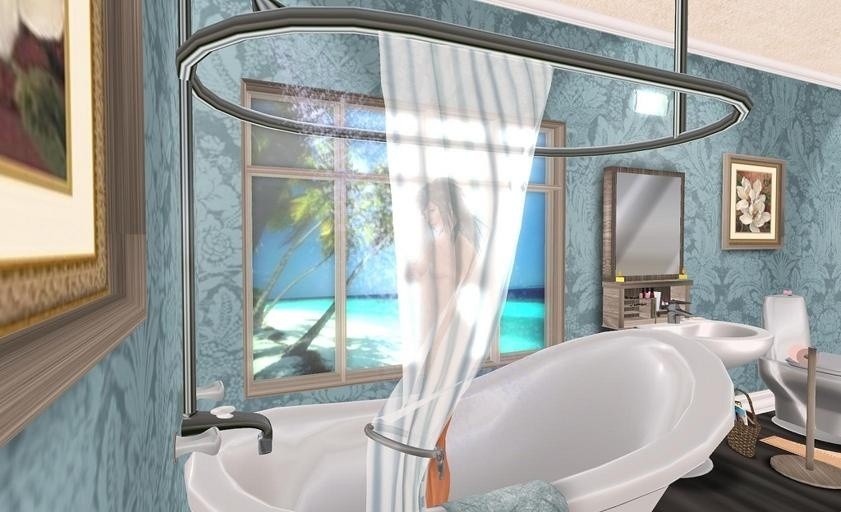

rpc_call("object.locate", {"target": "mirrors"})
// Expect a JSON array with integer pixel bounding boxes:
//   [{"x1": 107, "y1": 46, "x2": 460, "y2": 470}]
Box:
[{"x1": 603, "y1": 165, "x2": 686, "y2": 279}]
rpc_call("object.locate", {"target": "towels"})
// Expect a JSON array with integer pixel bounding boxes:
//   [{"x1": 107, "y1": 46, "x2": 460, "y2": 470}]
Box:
[{"x1": 439, "y1": 480, "x2": 570, "y2": 511}]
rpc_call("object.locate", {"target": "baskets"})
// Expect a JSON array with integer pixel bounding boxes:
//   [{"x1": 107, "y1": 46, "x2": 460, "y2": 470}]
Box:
[{"x1": 727, "y1": 387, "x2": 762, "y2": 459}]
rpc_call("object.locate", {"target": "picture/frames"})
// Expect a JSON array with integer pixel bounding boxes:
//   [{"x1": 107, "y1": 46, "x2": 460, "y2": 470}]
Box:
[
  {"x1": 721, "y1": 152, "x2": 787, "y2": 252},
  {"x1": 0, "y1": 0, "x2": 151, "y2": 451}
]
